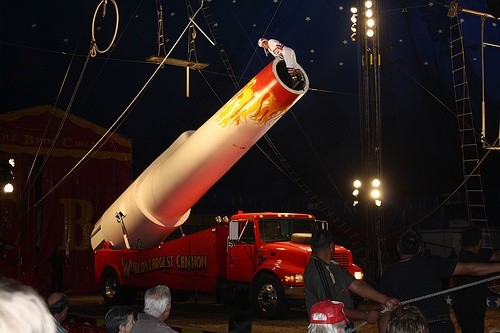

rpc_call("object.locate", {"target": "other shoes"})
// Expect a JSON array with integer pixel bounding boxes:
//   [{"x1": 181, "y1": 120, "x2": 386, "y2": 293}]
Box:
[{"x1": 292, "y1": 80, "x2": 305, "y2": 90}]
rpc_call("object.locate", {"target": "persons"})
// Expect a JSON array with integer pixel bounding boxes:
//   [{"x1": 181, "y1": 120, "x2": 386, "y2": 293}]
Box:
[
  {"x1": 0, "y1": 276, "x2": 180, "y2": 333},
  {"x1": 302, "y1": 229, "x2": 403, "y2": 333},
  {"x1": 378, "y1": 230, "x2": 500, "y2": 333},
  {"x1": 257, "y1": 37, "x2": 306, "y2": 91},
  {"x1": 307, "y1": 298, "x2": 429, "y2": 333},
  {"x1": 449, "y1": 224, "x2": 500, "y2": 333}
]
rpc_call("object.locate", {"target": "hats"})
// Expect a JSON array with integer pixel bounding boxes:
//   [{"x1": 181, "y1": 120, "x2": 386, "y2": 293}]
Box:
[
  {"x1": 258, "y1": 38, "x2": 268, "y2": 47},
  {"x1": 309, "y1": 300, "x2": 350, "y2": 324}
]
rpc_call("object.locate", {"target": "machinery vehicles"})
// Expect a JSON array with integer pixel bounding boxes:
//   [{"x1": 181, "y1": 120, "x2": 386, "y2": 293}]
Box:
[{"x1": 89, "y1": 57, "x2": 365, "y2": 320}]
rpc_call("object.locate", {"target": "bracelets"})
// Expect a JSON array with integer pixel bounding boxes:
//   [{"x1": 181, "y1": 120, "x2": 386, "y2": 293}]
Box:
[
  {"x1": 384, "y1": 298, "x2": 390, "y2": 305},
  {"x1": 365, "y1": 312, "x2": 368, "y2": 321}
]
